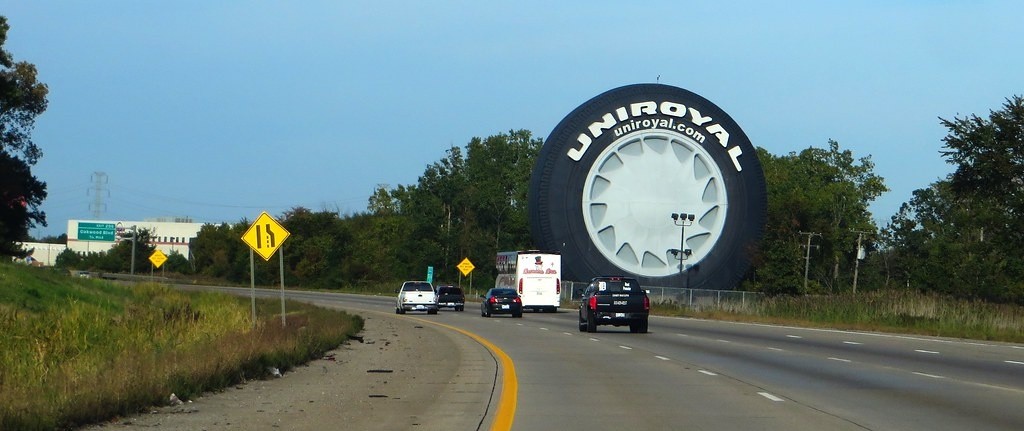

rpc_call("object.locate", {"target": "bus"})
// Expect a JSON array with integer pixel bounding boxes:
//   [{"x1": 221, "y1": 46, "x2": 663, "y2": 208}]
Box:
[{"x1": 494, "y1": 248, "x2": 562, "y2": 312}]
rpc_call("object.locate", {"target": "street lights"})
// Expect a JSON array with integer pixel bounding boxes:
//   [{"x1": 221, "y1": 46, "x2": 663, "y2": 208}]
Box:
[{"x1": 668, "y1": 212, "x2": 695, "y2": 272}]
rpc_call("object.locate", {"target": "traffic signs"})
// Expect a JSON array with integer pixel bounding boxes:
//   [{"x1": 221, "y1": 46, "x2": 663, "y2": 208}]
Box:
[{"x1": 78, "y1": 222, "x2": 116, "y2": 242}]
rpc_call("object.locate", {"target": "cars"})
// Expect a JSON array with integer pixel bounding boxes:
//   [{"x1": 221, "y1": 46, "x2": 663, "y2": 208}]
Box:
[
  {"x1": 578, "y1": 276, "x2": 650, "y2": 333},
  {"x1": 76, "y1": 272, "x2": 92, "y2": 279},
  {"x1": 479, "y1": 288, "x2": 523, "y2": 317}
]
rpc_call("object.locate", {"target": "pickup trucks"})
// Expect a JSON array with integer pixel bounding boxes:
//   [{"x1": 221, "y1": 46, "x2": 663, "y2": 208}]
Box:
[
  {"x1": 396, "y1": 281, "x2": 438, "y2": 315},
  {"x1": 436, "y1": 286, "x2": 465, "y2": 312},
  {"x1": 12, "y1": 256, "x2": 44, "y2": 267}
]
